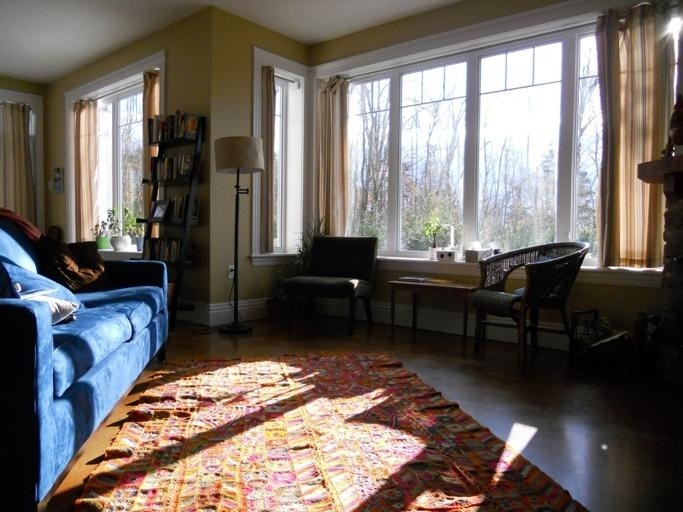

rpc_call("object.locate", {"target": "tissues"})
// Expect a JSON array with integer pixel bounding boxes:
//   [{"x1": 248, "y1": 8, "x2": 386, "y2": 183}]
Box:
[{"x1": 465, "y1": 241, "x2": 491, "y2": 263}]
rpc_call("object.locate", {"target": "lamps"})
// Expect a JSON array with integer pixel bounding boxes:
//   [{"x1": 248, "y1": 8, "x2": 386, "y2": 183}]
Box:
[{"x1": 213, "y1": 135, "x2": 266, "y2": 336}]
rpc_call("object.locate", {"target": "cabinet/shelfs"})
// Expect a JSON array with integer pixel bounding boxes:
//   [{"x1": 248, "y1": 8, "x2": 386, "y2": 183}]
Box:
[{"x1": 135, "y1": 112, "x2": 206, "y2": 282}]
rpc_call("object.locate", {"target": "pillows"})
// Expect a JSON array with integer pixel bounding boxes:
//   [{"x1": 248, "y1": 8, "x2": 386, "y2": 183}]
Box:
[{"x1": 0, "y1": 203, "x2": 118, "y2": 326}]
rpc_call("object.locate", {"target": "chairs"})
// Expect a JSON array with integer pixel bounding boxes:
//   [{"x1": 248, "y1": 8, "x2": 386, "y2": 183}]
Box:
[
  {"x1": 469, "y1": 241, "x2": 591, "y2": 383},
  {"x1": 276, "y1": 235, "x2": 381, "y2": 337}
]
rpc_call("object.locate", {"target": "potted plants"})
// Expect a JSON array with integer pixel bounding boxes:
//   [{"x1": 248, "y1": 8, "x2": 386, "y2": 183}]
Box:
[{"x1": 91, "y1": 209, "x2": 144, "y2": 251}]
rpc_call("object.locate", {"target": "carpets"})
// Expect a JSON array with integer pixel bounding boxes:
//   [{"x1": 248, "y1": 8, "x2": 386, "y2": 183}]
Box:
[{"x1": 76, "y1": 347, "x2": 588, "y2": 512}]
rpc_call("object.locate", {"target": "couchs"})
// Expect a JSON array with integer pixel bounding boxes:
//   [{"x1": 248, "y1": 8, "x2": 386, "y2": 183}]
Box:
[{"x1": 0, "y1": 220, "x2": 171, "y2": 510}]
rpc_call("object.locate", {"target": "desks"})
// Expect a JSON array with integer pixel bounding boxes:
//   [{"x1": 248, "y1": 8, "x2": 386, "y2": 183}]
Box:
[{"x1": 387, "y1": 277, "x2": 488, "y2": 350}]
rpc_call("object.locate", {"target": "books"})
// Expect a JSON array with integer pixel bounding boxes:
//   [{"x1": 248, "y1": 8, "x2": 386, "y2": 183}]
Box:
[{"x1": 140, "y1": 109, "x2": 202, "y2": 263}]
